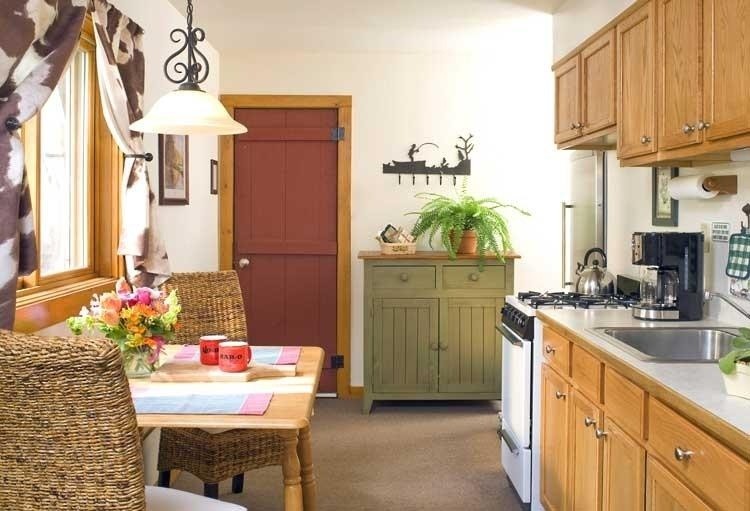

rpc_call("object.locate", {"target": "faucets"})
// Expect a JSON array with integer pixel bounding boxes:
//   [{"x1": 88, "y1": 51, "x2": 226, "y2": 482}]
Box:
[{"x1": 703, "y1": 290, "x2": 750, "y2": 320}]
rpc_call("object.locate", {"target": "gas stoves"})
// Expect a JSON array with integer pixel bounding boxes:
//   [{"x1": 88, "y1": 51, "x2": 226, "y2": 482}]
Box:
[{"x1": 499, "y1": 290, "x2": 636, "y2": 307}]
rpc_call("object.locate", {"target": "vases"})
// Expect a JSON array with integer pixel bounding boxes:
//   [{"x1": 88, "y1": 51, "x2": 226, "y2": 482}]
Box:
[{"x1": 121, "y1": 347, "x2": 155, "y2": 379}]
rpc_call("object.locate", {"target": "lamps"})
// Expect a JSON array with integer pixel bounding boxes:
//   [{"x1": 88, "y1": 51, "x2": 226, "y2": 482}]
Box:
[{"x1": 128, "y1": 0, "x2": 248, "y2": 138}]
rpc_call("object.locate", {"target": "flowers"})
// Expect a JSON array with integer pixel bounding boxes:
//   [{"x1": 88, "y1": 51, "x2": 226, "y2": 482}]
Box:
[{"x1": 66, "y1": 278, "x2": 181, "y2": 371}]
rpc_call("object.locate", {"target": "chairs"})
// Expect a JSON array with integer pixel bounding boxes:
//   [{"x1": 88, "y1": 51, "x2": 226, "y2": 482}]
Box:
[
  {"x1": 153, "y1": 272, "x2": 285, "y2": 497},
  {"x1": 1, "y1": 328, "x2": 248, "y2": 510}
]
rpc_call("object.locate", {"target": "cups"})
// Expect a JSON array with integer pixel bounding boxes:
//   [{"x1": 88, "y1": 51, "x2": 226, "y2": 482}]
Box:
[
  {"x1": 218, "y1": 341, "x2": 252, "y2": 372},
  {"x1": 199, "y1": 334, "x2": 229, "y2": 366}
]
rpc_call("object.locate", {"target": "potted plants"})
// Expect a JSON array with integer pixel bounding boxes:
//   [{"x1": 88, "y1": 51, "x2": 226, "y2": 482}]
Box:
[
  {"x1": 718, "y1": 328, "x2": 750, "y2": 399},
  {"x1": 405, "y1": 192, "x2": 530, "y2": 272}
]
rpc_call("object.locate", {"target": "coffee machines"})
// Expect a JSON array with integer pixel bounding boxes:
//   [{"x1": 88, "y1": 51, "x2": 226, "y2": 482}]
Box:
[{"x1": 631, "y1": 231, "x2": 705, "y2": 322}]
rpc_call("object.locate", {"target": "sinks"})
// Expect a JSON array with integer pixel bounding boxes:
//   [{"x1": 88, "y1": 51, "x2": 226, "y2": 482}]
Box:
[{"x1": 585, "y1": 325, "x2": 744, "y2": 364}]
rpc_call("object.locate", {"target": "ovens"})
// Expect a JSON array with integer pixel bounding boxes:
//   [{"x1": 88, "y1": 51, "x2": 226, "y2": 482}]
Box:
[{"x1": 492, "y1": 323, "x2": 531, "y2": 506}]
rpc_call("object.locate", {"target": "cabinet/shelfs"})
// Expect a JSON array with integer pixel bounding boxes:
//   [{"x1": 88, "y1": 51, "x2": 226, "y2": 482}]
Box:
[
  {"x1": 550, "y1": 17, "x2": 617, "y2": 152},
  {"x1": 616, "y1": 0, "x2": 657, "y2": 171},
  {"x1": 658, "y1": 0, "x2": 750, "y2": 167},
  {"x1": 571, "y1": 329, "x2": 646, "y2": 511},
  {"x1": 358, "y1": 250, "x2": 522, "y2": 416},
  {"x1": 534, "y1": 309, "x2": 570, "y2": 511},
  {"x1": 647, "y1": 374, "x2": 750, "y2": 511}
]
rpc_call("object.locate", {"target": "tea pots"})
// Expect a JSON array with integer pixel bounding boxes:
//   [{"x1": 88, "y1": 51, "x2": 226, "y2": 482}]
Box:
[{"x1": 575, "y1": 248, "x2": 616, "y2": 300}]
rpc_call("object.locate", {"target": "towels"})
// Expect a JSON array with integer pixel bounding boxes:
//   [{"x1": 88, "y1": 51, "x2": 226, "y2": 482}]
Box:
[
  {"x1": 167, "y1": 347, "x2": 302, "y2": 366},
  {"x1": 133, "y1": 391, "x2": 274, "y2": 415}
]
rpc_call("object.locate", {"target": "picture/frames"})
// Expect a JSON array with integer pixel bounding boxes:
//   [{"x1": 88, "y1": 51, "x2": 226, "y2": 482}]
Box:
[
  {"x1": 158, "y1": 133, "x2": 190, "y2": 206},
  {"x1": 653, "y1": 166, "x2": 679, "y2": 227}
]
